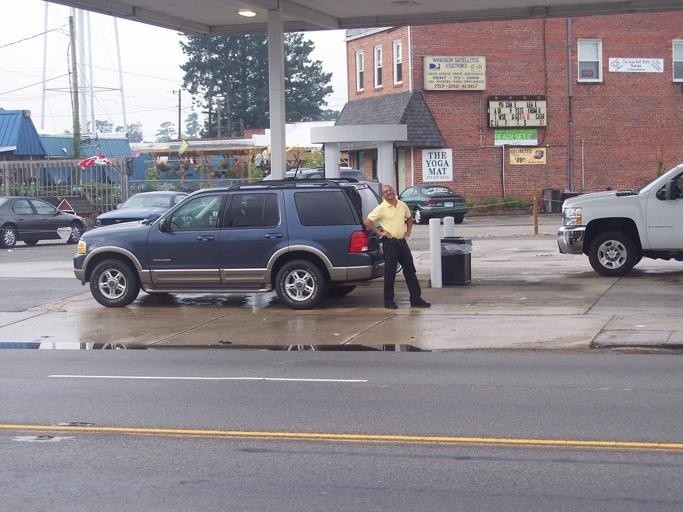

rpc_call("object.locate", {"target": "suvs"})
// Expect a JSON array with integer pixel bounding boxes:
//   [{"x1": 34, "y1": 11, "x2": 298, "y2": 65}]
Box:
[
  {"x1": 72, "y1": 176, "x2": 402, "y2": 307},
  {"x1": 262, "y1": 166, "x2": 367, "y2": 181}
]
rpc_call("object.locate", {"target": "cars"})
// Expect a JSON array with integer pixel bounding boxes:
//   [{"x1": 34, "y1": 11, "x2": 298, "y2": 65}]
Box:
[
  {"x1": 557, "y1": 163, "x2": 683, "y2": 277},
  {"x1": 396, "y1": 184, "x2": 470, "y2": 225},
  {"x1": 0, "y1": 196, "x2": 87, "y2": 249},
  {"x1": 94, "y1": 191, "x2": 188, "y2": 229}
]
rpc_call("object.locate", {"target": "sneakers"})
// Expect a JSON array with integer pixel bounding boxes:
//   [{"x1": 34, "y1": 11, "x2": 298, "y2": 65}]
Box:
[
  {"x1": 384, "y1": 301, "x2": 397, "y2": 309},
  {"x1": 410, "y1": 297, "x2": 430, "y2": 307}
]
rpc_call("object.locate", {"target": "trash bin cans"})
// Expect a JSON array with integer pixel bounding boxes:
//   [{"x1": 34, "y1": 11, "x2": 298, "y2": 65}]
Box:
[
  {"x1": 441, "y1": 236, "x2": 471, "y2": 285},
  {"x1": 543, "y1": 188, "x2": 578, "y2": 213}
]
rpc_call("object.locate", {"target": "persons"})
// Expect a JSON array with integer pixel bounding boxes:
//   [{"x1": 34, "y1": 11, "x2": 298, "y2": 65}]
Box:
[{"x1": 364, "y1": 185, "x2": 431, "y2": 309}]
